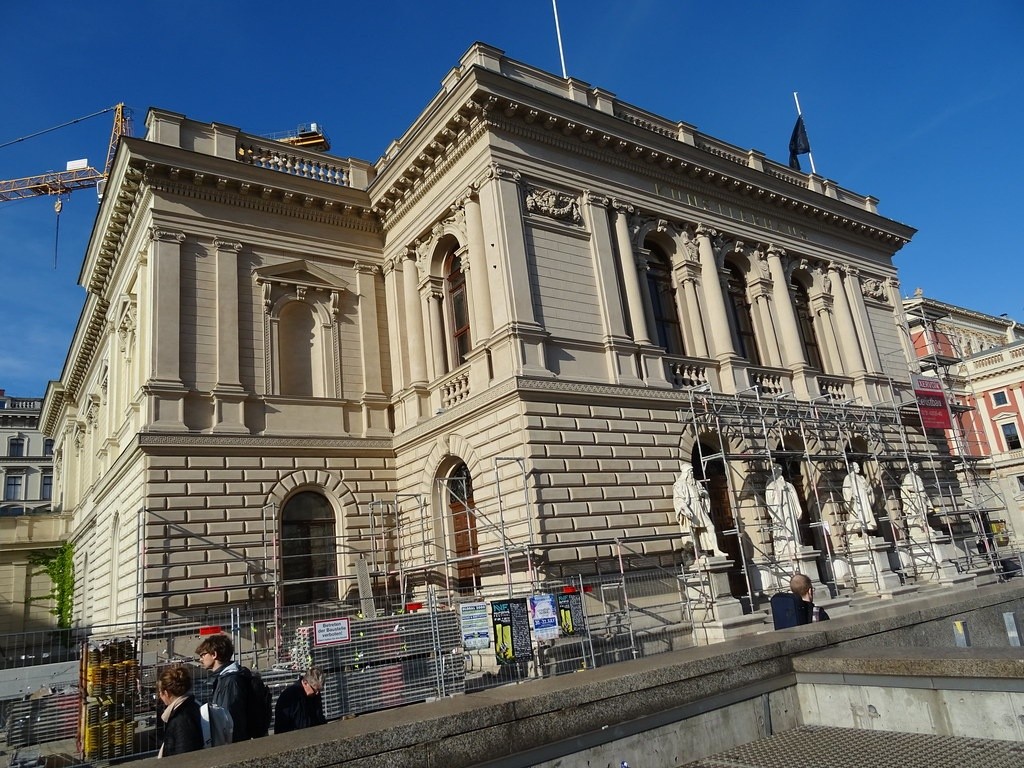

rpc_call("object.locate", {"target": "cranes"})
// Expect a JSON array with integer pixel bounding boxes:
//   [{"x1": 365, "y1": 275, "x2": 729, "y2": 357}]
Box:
[{"x1": 0, "y1": 103, "x2": 334, "y2": 201}]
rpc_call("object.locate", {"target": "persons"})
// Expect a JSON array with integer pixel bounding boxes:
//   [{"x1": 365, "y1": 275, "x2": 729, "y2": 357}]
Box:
[
  {"x1": 672, "y1": 462, "x2": 729, "y2": 558},
  {"x1": 157, "y1": 668, "x2": 205, "y2": 759},
  {"x1": 765, "y1": 462, "x2": 805, "y2": 555},
  {"x1": 195, "y1": 635, "x2": 273, "y2": 745},
  {"x1": 789, "y1": 574, "x2": 831, "y2": 626},
  {"x1": 273, "y1": 667, "x2": 329, "y2": 735},
  {"x1": 900, "y1": 459, "x2": 935, "y2": 537},
  {"x1": 842, "y1": 461, "x2": 879, "y2": 533}
]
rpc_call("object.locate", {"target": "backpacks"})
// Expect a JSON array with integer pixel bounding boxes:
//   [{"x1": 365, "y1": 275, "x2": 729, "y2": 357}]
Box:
[
  {"x1": 220, "y1": 667, "x2": 273, "y2": 739},
  {"x1": 771, "y1": 593, "x2": 807, "y2": 630}
]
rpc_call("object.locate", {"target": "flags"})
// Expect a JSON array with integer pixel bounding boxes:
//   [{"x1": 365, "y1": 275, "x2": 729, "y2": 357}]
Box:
[{"x1": 788, "y1": 114, "x2": 811, "y2": 169}]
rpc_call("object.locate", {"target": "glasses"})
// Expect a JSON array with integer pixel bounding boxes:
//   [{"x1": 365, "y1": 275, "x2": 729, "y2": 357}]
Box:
[
  {"x1": 807, "y1": 586, "x2": 816, "y2": 594},
  {"x1": 305, "y1": 679, "x2": 320, "y2": 695},
  {"x1": 200, "y1": 652, "x2": 208, "y2": 658}
]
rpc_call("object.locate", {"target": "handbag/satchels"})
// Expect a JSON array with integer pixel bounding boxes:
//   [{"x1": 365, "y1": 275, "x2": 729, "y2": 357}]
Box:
[{"x1": 194, "y1": 698, "x2": 234, "y2": 749}]
